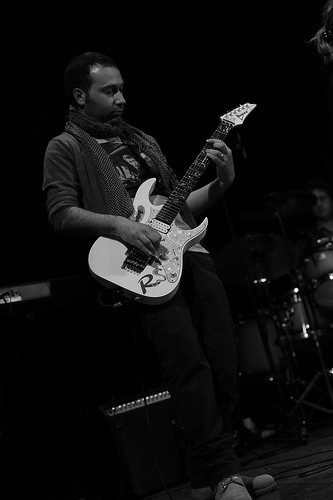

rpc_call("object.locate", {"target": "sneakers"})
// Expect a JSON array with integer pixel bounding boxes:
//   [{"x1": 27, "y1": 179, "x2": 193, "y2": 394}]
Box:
[
  {"x1": 212, "y1": 471, "x2": 252, "y2": 500},
  {"x1": 189, "y1": 473, "x2": 278, "y2": 500}
]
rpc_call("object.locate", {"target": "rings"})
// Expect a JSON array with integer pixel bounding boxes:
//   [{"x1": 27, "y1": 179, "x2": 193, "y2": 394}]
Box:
[{"x1": 220, "y1": 153, "x2": 224, "y2": 158}]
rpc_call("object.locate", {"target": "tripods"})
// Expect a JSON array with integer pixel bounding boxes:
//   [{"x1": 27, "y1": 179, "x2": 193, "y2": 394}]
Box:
[{"x1": 245, "y1": 205, "x2": 333, "y2": 444}]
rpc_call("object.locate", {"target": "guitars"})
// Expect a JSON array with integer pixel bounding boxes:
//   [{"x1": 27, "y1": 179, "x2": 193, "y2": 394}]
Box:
[{"x1": 88, "y1": 102, "x2": 257, "y2": 304}]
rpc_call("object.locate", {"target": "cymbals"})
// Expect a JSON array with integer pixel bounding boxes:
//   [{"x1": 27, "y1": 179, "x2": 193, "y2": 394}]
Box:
[
  {"x1": 214, "y1": 232, "x2": 301, "y2": 284},
  {"x1": 239, "y1": 190, "x2": 317, "y2": 221}
]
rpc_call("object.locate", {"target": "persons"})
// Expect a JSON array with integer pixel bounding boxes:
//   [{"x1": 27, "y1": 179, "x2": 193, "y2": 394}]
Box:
[
  {"x1": 298, "y1": 186, "x2": 333, "y2": 266},
  {"x1": 44, "y1": 52, "x2": 276, "y2": 500}
]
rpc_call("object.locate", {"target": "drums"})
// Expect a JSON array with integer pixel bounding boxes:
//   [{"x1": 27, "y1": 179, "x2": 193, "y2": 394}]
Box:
[
  {"x1": 272, "y1": 290, "x2": 333, "y2": 348},
  {"x1": 232, "y1": 310, "x2": 290, "y2": 376},
  {"x1": 302, "y1": 239, "x2": 333, "y2": 307}
]
